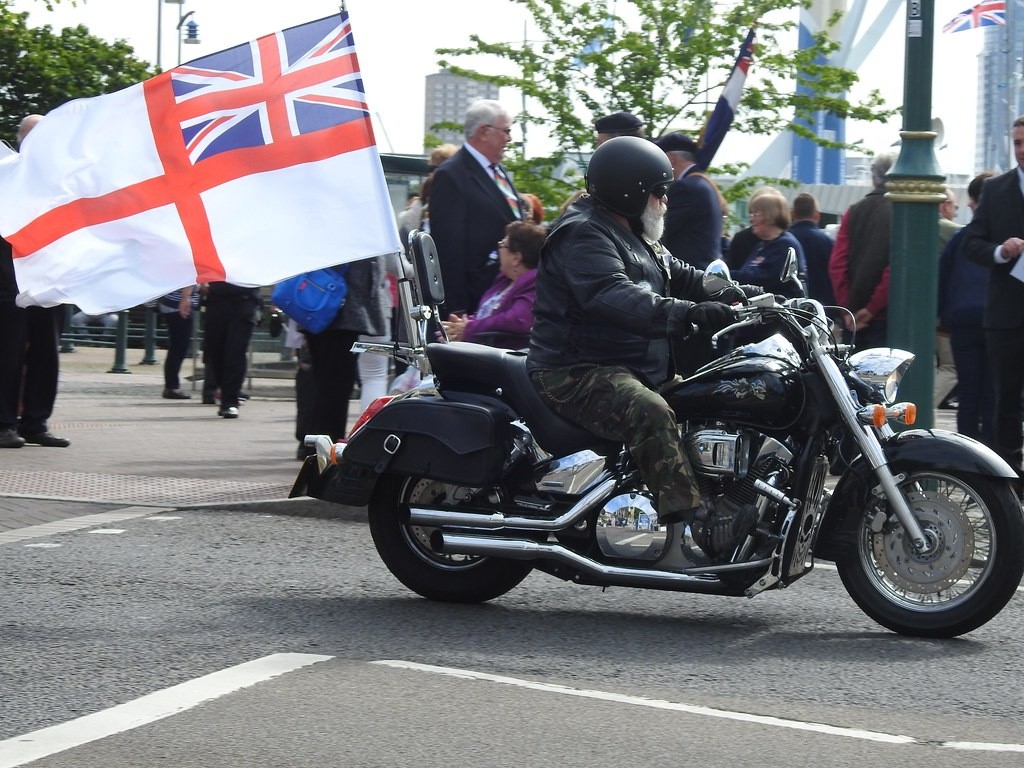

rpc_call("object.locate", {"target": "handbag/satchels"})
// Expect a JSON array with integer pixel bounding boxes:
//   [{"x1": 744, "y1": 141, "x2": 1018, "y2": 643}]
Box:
[{"x1": 272, "y1": 267, "x2": 347, "y2": 335}]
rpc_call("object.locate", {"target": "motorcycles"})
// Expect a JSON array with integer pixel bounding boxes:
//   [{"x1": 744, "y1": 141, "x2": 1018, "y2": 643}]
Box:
[{"x1": 285, "y1": 229, "x2": 1024, "y2": 639}]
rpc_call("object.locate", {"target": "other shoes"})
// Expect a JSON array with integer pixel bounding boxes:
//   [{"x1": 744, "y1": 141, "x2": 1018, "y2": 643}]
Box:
[
  {"x1": 218, "y1": 407, "x2": 239, "y2": 418},
  {"x1": 162, "y1": 389, "x2": 190, "y2": 399},
  {"x1": 297, "y1": 441, "x2": 316, "y2": 460}
]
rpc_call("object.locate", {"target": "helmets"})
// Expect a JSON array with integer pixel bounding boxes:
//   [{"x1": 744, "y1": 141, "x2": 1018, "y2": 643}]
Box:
[{"x1": 584, "y1": 136, "x2": 674, "y2": 219}]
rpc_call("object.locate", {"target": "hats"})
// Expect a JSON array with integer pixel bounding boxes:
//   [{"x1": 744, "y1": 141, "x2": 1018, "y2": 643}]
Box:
[
  {"x1": 595, "y1": 112, "x2": 643, "y2": 133},
  {"x1": 653, "y1": 133, "x2": 698, "y2": 153}
]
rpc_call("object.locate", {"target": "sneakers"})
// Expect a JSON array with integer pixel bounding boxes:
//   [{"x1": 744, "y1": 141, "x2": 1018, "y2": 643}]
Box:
[
  {"x1": 692, "y1": 500, "x2": 759, "y2": 566},
  {"x1": 0, "y1": 428, "x2": 26, "y2": 448},
  {"x1": 22, "y1": 431, "x2": 70, "y2": 447}
]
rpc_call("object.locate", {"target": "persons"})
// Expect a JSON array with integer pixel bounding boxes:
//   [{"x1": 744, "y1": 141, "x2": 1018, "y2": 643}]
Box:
[
  {"x1": 526, "y1": 136, "x2": 763, "y2": 552},
  {"x1": 716, "y1": 114, "x2": 1024, "y2": 499},
  {"x1": 0, "y1": 115, "x2": 72, "y2": 450},
  {"x1": 387, "y1": 99, "x2": 733, "y2": 403},
  {"x1": 294, "y1": 256, "x2": 388, "y2": 460},
  {"x1": 73, "y1": 281, "x2": 363, "y2": 419}
]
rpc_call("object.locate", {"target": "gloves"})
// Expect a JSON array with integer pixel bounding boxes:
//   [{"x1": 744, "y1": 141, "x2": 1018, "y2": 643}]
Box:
[{"x1": 689, "y1": 301, "x2": 733, "y2": 331}]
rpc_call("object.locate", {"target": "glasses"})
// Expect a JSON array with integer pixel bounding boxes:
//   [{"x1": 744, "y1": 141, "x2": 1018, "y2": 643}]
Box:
[
  {"x1": 498, "y1": 241, "x2": 521, "y2": 251},
  {"x1": 485, "y1": 125, "x2": 511, "y2": 135},
  {"x1": 748, "y1": 209, "x2": 761, "y2": 216},
  {"x1": 650, "y1": 184, "x2": 668, "y2": 200}
]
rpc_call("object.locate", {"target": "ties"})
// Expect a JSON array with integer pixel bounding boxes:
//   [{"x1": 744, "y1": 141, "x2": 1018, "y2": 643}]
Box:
[{"x1": 488, "y1": 163, "x2": 521, "y2": 220}]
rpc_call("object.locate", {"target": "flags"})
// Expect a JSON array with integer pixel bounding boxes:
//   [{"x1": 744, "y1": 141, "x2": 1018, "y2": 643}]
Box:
[
  {"x1": 0, "y1": 12, "x2": 402, "y2": 315},
  {"x1": 942, "y1": 0, "x2": 1007, "y2": 34}
]
